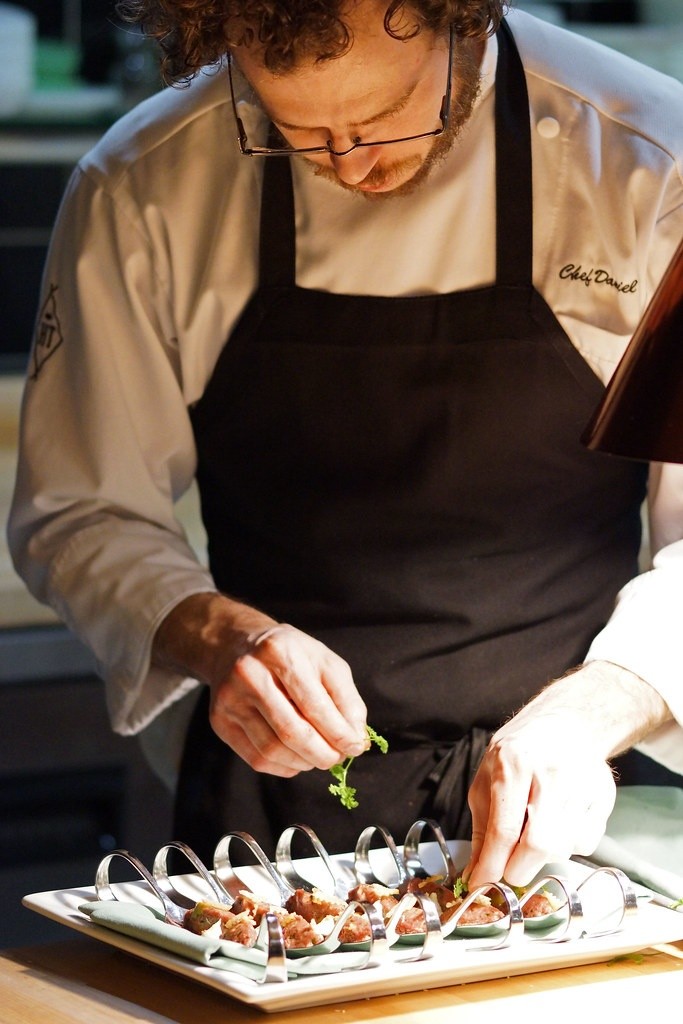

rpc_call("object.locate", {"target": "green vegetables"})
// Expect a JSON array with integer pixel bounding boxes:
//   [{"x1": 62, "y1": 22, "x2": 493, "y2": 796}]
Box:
[
  {"x1": 453, "y1": 878, "x2": 468, "y2": 898},
  {"x1": 328, "y1": 725, "x2": 388, "y2": 808}
]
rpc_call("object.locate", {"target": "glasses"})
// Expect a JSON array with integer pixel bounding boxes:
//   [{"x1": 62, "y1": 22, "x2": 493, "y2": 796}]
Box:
[{"x1": 223, "y1": 8, "x2": 457, "y2": 156}]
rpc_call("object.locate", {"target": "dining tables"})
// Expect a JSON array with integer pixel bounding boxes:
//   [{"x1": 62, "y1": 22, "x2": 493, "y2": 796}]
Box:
[{"x1": 0, "y1": 943, "x2": 683, "y2": 1024}]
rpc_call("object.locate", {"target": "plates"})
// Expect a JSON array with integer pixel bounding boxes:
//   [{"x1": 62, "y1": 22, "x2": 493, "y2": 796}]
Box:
[{"x1": 22, "y1": 837, "x2": 683, "y2": 1016}]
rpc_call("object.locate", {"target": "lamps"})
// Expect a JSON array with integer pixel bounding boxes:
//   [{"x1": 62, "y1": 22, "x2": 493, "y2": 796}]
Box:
[{"x1": 0, "y1": 39, "x2": 121, "y2": 164}]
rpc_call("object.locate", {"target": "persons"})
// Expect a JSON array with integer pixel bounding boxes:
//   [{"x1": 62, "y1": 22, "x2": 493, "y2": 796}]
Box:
[{"x1": 5, "y1": 0, "x2": 683, "y2": 896}]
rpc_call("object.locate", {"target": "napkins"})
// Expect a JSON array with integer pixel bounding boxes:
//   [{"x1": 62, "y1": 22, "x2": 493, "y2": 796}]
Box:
[{"x1": 592, "y1": 785, "x2": 683, "y2": 901}]
